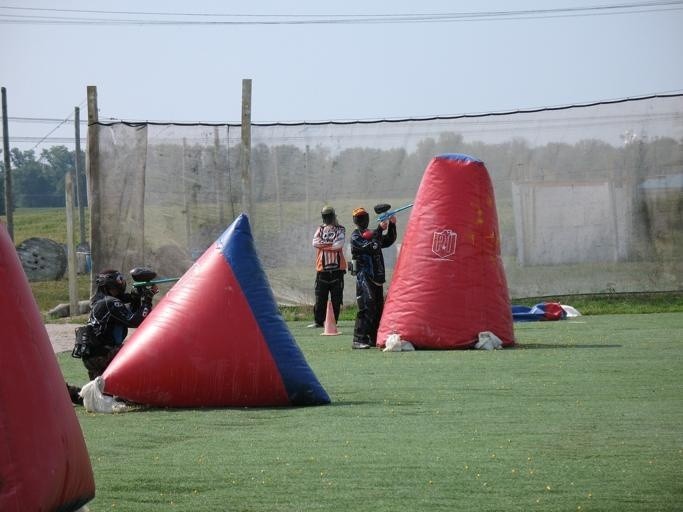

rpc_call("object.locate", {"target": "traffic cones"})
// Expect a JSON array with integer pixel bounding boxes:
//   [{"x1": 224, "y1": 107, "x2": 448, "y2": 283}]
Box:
[{"x1": 319, "y1": 298, "x2": 343, "y2": 336}]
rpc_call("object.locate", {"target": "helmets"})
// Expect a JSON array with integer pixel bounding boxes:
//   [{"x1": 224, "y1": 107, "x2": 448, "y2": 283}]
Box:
[
  {"x1": 95, "y1": 270, "x2": 127, "y2": 296},
  {"x1": 321, "y1": 206, "x2": 335, "y2": 224},
  {"x1": 545, "y1": 304, "x2": 567, "y2": 320},
  {"x1": 353, "y1": 208, "x2": 369, "y2": 228}
]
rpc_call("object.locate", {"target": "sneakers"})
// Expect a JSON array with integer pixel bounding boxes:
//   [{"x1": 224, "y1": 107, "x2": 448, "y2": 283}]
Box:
[
  {"x1": 352, "y1": 341, "x2": 370, "y2": 349},
  {"x1": 307, "y1": 323, "x2": 322, "y2": 328},
  {"x1": 66, "y1": 382, "x2": 76, "y2": 398}
]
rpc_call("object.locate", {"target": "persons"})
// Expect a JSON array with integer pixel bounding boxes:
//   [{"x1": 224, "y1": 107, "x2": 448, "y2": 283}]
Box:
[
  {"x1": 63, "y1": 268, "x2": 159, "y2": 404},
  {"x1": 350, "y1": 207, "x2": 396, "y2": 349},
  {"x1": 307, "y1": 205, "x2": 346, "y2": 327}
]
